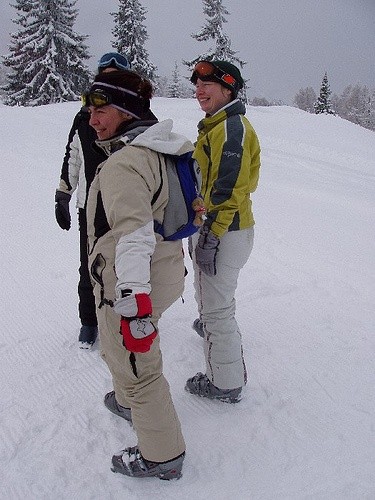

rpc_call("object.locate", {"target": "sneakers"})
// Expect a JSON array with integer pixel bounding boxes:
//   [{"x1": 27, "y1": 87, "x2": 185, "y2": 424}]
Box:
[
  {"x1": 192, "y1": 319, "x2": 205, "y2": 337},
  {"x1": 105, "y1": 392, "x2": 132, "y2": 421},
  {"x1": 111, "y1": 444, "x2": 186, "y2": 481},
  {"x1": 185, "y1": 372, "x2": 242, "y2": 403},
  {"x1": 79, "y1": 326, "x2": 98, "y2": 350}
]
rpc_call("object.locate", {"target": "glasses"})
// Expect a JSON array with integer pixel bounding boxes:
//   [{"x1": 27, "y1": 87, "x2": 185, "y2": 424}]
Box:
[
  {"x1": 100, "y1": 52, "x2": 129, "y2": 67},
  {"x1": 80, "y1": 93, "x2": 111, "y2": 109},
  {"x1": 195, "y1": 60, "x2": 215, "y2": 76}
]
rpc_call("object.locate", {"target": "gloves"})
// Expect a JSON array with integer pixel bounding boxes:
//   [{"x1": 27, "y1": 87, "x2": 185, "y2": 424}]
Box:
[
  {"x1": 113, "y1": 292, "x2": 156, "y2": 354},
  {"x1": 195, "y1": 228, "x2": 219, "y2": 277},
  {"x1": 54, "y1": 200, "x2": 71, "y2": 231}
]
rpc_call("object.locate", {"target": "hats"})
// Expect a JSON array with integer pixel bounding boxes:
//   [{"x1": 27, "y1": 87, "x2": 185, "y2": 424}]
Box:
[
  {"x1": 92, "y1": 70, "x2": 150, "y2": 120},
  {"x1": 191, "y1": 60, "x2": 244, "y2": 93},
  {"x1": 97, "y1": 53, "x2": 131, "y2": 72}
]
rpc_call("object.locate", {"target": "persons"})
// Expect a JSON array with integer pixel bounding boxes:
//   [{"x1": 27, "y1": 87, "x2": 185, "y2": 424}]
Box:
[
  {"x1": 80, "y1": 71, "x2": 186, "y2": 482},
  {"x1": 184, "y1": 61, "x2": 262, "y2": 405},
  {"x1": 54, "y1": 52, "x2": 130, "y2": 349}
]
